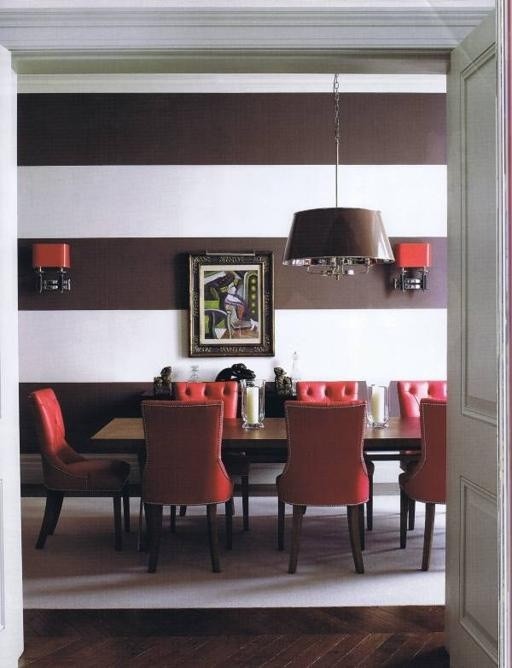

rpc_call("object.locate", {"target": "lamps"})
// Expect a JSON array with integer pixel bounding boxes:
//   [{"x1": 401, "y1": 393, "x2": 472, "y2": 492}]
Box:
[
  {"x1": 394, "y1": 244, "x2": 432, "y2": 292},
  {"x1": 281, "y1": 75, "x2": 396, "y2": 279},
  {"x1": 31, "y1": 245, "x2": 72, "y2": 296}
]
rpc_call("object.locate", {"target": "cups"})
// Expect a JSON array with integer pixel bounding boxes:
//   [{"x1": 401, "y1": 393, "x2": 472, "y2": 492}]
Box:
[
  {"x1": 367, "y1": 384, "x2": 389, "y2": 428},
  {"x1": 240, "y1": 378, "x2": 266, "y2": 429}
]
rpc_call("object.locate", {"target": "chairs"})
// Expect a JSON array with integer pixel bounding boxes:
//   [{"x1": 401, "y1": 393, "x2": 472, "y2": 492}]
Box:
[
  {"x1": 297, "y1": 380, "x2": 374, "y2": 531},
  {"x1": 176, "y1": 381, "x2": 250, "y2": 529},
  {"x1": 30, "y1": 387, "x2": 132, "y2": 550},
  {"x1": 278, "y1": 401, "x2": 365, "y2": 574},
  {"x1": 397, "y1": 380, "x2": 415, "y2": 529},
  {"x1": 139, "y1": 399, "x2": 234, "y2": 573}
]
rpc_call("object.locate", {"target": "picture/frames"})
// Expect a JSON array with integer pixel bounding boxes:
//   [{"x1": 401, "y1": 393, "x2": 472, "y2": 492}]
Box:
[{"x1": 186, "y1": 249, "x2": 276, "y2": 358}]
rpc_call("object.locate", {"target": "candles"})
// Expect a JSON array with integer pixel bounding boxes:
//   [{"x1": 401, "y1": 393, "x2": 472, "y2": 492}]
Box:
[
  {"x1": 373, "y1": 385, "x2": 384, "y2": 422},
  {"x1": 247, "y1": 386, "x2": 260, "y2": 423}
]
